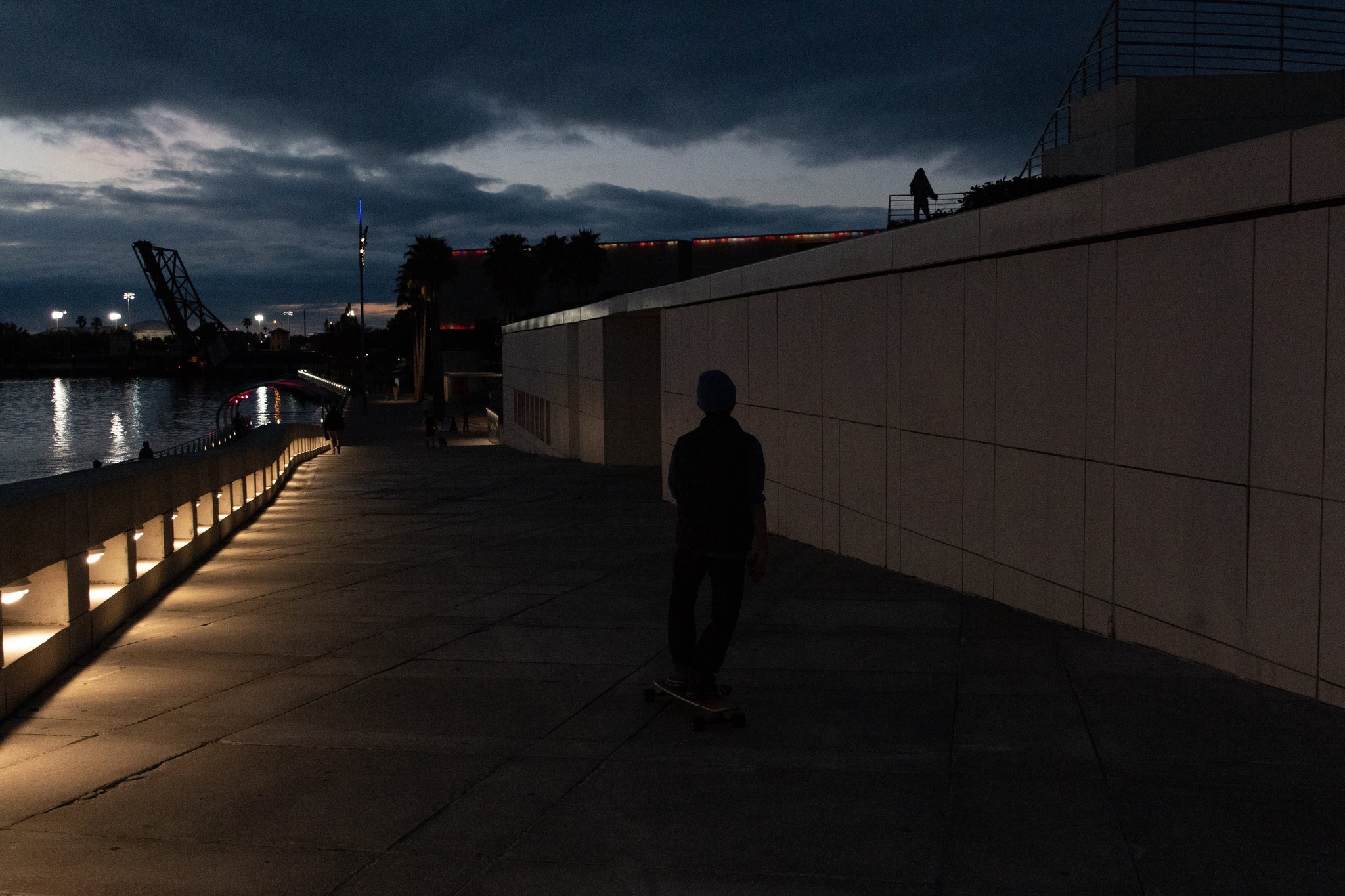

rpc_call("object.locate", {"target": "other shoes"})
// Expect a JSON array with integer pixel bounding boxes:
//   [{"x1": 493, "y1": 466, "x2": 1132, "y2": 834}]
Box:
[
  {"x1": 666, "y1": 663, "x2": 687, "y2": 686},
  {"x1": 685, "y1": 670, "x2": 732, "y2": 701}
]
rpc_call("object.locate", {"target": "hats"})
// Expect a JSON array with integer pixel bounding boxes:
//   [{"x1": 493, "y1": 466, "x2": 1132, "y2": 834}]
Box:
[{"x1": 697, "y1": 371, "x2": 736, "y2": 412}]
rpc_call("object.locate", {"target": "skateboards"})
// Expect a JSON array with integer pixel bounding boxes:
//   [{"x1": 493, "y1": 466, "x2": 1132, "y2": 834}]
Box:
[{"x1": 641, "y1": 675, "x2": 748, "y2": 731}]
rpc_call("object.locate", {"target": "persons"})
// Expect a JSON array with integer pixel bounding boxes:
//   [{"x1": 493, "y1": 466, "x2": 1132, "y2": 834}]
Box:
[
  {"x1": 489, "y1": 393, "x2": 492, "y2": 409},
  {"x1": 667, "y1": 370, "x2": 768, "y2": 701},
  {"x1": 138, "y1": 441, "x2": 154, "y2": 464},
  {"x1": 422, "y1": 414, "x2": 439, "y2": 448},
  {"x1": 462, "y1": 401, "x2": 470, "y2": 433},
  {"x1": 323, "y1": 407, "x2": 345, "y2": 455},
  {"x1": 909, "y1": 167, "x2": 939, "y2": 220},
  {"x1": 422, "y1": 398, "x2": 430, "y2": 421},
  {"x1": 393, "y1": 375, "x2": 400, "y2": 401},
  {"x1": 233, "y1": 412, "x2": 249, "y2": 438}
]
